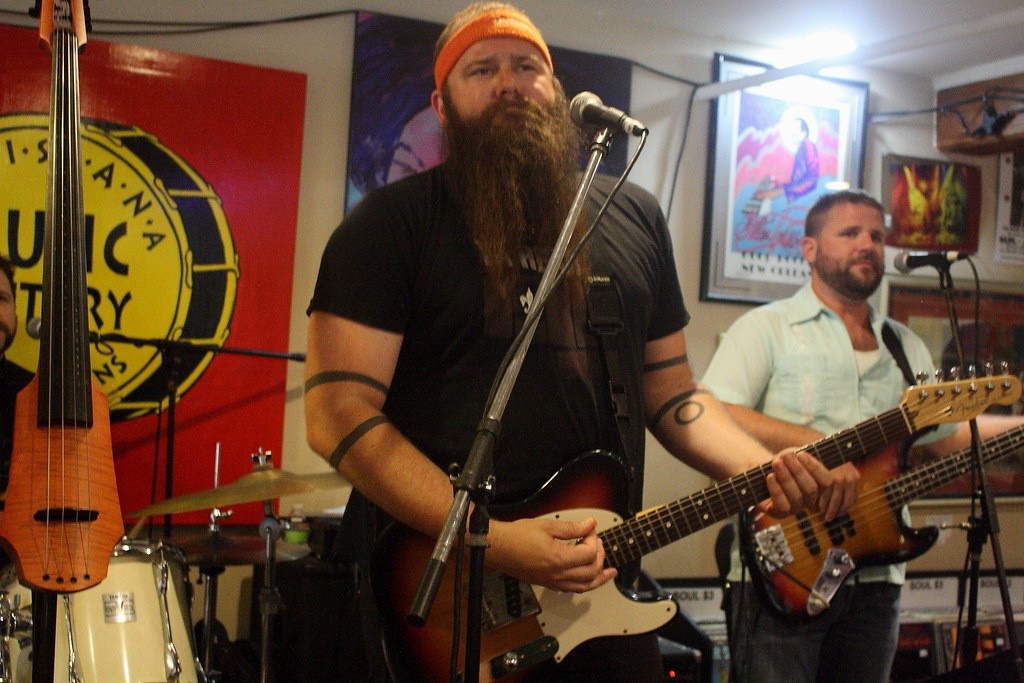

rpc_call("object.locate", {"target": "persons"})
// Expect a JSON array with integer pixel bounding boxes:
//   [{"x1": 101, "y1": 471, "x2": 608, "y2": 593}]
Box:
[
  {"x1": 302, "y1": 1, "x2": 833, "y2": 683},
  {"x1": 695, "y1": 190, "x2": 1024, "y2": 683},
  {"x1": 0, "y1": 255, "x2": 33, "y2": 570}
]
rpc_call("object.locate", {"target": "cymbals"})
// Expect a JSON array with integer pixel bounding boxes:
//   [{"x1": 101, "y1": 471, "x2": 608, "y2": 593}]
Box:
[
  {"x1": 157, "y1": 528, "x2": 312, "y2": 567},
  {"x1": 134, "y1": 467, "x2": 355, "y2": 519}
]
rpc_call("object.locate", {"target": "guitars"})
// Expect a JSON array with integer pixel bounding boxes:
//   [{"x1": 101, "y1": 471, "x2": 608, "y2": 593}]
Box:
[
  {"x1": 742, "y1": 421, "x2": 1024, "y2": 618},
  {"x1": 0, "y1": 0, "x2": 126, "y2": 596},
  {"x1": 381, "y1": 361, "x2": 1022, "y2": 683}
]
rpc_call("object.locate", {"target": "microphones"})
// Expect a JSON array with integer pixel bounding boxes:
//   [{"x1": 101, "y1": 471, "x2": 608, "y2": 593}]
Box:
[
  {"x1": 27, "y1": 317, "x2": 102, "y2": 343},
  {"x1": 569, "y1": 91, "x2": 649, "y2": 136},
  {"x1": 894, "y1": 251, "x2": 967, "y2": 272}
]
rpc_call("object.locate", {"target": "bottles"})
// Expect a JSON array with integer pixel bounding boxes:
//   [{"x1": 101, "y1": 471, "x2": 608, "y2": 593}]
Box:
[{"x1": 285, "y1": 503, "x2": 309, "y2": 543}]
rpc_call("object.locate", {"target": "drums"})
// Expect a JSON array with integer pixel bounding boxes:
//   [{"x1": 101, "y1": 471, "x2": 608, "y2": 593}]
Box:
[{"x1": 0, "y1": 539, "x2": 203, "y2": 683}]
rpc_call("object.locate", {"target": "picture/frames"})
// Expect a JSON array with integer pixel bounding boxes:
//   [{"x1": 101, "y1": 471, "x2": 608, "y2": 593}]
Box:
[{"x1": 697, "y1": 52, "x2": 871, "y2": 305}]
